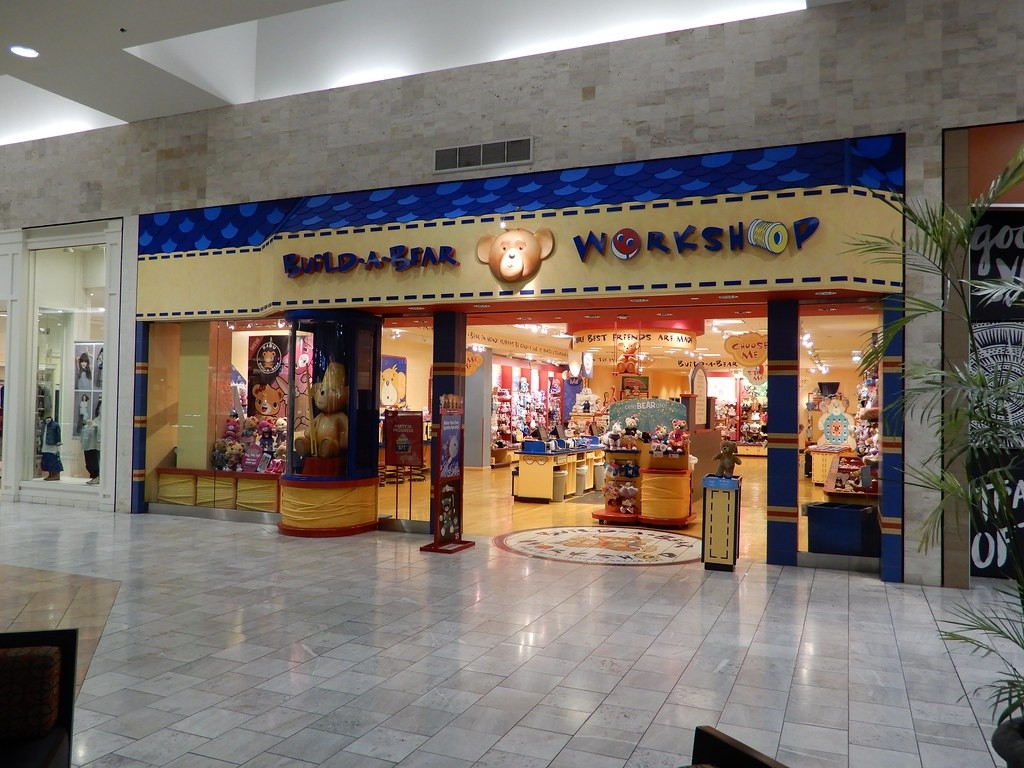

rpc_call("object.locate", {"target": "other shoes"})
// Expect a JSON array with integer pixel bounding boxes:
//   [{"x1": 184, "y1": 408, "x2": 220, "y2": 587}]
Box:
[{"x1": 86, "y1": 476, "x2": 99, "y2": 484}]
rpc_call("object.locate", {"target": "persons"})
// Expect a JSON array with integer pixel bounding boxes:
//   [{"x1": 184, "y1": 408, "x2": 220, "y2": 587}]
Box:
[
  {"x1": 76, "y1": 348, "x2": 103, "y2": 433},
  {"x1": 40, "y1": 417, "x2": 100, "y2": 484}
]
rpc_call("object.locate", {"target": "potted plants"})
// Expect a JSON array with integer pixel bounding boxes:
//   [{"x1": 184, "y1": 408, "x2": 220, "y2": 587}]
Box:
[{"x1": 836, "y1": 143, "x2": 1024, "y2": 768}]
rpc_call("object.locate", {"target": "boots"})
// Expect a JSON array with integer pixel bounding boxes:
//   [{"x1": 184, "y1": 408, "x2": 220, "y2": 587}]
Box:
[{"x1": 44, "y1": 471, "x2": 60, "y2": 480}]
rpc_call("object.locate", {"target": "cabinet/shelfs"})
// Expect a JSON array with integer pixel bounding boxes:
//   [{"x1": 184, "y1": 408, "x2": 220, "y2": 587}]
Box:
[{"x1": 490, "y1": 356, "x2": 880, "y2": 526}]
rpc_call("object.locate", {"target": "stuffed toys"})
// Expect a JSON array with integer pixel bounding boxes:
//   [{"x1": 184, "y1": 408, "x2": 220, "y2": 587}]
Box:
[
  {"x1": 211, "y1": 411, "x2": 286, "y2": 474},
  {"x1": 294, "y1": 361, "x2": 349, "y2": 458},
  {"x1": 490, "y1": 340, "x2": 879, "y2": 513}
]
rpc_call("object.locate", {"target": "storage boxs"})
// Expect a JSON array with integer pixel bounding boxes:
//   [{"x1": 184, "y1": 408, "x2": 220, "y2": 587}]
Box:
[{"x1": 808, "y1": 503, "x2": 877, "y2": 557}]
